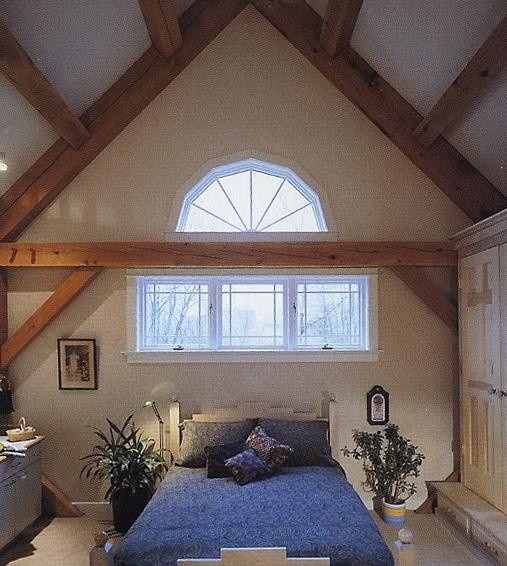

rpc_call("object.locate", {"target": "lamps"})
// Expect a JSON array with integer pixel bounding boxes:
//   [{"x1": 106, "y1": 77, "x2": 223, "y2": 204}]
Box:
[{"x1": 143, "y1": 381, "x2": 182, "y2": 457}]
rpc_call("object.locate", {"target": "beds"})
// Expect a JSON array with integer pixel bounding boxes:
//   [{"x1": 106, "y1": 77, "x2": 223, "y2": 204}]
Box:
[{"x1": 89, "y1": 393, "x2": 416, "y2": 566}]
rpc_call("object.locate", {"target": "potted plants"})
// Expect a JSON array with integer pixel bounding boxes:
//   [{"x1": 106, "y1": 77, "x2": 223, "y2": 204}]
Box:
[
  {"x1": 340, "y1": 423, "x2": 426, "y2": 526},
  {"x1": 79, "y1": 414, "x2": 174, "y2": 534}
]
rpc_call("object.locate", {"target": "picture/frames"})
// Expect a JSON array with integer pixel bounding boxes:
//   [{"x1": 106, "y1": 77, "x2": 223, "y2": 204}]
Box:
[{"x1": 57, "y1": 338, "x2": 97, "y2": 391}]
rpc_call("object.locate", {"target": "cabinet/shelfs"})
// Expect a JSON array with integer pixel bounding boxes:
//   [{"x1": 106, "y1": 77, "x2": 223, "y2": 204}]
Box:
[
  {"x1": 0, "y1": 436, "x2": 47, "y2": 549},
  {"x1": 426, "y1": 209, "x2": 506, "y2": 565}
]
